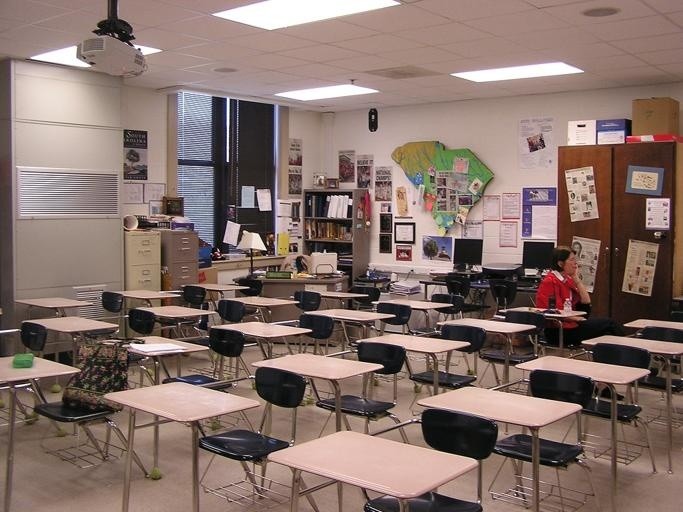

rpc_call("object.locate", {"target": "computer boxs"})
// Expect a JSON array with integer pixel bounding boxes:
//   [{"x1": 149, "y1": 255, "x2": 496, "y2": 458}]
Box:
[
  {"x1": 448, "y1": 271, "x2": 481, "y2": 282},
  {"x1": 517, "y1": 276, "x2": 541, "y2": 287}
]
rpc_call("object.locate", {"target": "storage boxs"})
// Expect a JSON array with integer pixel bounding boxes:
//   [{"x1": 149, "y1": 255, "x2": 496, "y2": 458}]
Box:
[
  {"x1": 630, "y1": 97, "x2": 679, "y2": 138},
  {"x1": 596, "y1": 119, "x2": 631, "y2": 145},
  {"x1": 566, "y1": 120, "x2": 596, "y2": 146}
]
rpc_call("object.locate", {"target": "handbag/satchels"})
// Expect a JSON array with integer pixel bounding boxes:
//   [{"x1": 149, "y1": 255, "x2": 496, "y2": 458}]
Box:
[{"x1": 61, "y1": 340, "x2": 129, "y2": 412}]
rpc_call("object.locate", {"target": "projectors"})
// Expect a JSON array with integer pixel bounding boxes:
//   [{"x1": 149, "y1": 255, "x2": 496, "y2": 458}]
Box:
[{"x1": 77, "y1": 35, "x2": 148, "y2": 78}]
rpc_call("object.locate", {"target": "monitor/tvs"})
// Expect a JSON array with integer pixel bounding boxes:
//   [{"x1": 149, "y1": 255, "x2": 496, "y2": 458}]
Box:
[
  {"x1": 453, "y1": 238, "x2": 483, "y2": 272},
  {"x1": 522, "y1": 241, "x2": 555, "y2": 275},
  {"x1": 310, "y1": 252, "x2": 338, "y2": 274}
]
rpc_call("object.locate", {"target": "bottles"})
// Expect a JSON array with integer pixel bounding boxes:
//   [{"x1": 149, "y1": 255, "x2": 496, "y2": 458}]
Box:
[{"x1": 563, "y1": 298, "x2": 573, "y2": 316}]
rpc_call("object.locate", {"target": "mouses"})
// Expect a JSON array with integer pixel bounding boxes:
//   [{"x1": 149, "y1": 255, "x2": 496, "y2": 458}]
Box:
[
  {"x1": 533, "y1": 285, "x2": 538, "y2": 289},
  {"x1": 476, "y1": 280, "x2": 481, "y2": 285}
]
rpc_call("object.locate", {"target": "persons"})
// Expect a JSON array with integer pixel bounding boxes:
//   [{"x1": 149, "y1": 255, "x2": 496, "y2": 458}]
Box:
[{"x1": 537, "y1": 247, "x2": 648, "y2": 400}]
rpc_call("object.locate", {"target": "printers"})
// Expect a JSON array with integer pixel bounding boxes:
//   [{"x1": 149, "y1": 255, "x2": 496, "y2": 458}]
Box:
[{"x1": 481, "y1": 263, "x2": 523, "y2": 283}]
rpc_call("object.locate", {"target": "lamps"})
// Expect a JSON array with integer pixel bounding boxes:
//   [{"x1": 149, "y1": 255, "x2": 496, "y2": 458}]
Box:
[{"x1": 236, "y1": 232, "x2": 267, "y2": 279}]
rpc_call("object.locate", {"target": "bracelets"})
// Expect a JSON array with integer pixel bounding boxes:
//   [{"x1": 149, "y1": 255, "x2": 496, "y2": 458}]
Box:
[{"x1": 573, "y1": 277, "x2": 581, "y2": 284}]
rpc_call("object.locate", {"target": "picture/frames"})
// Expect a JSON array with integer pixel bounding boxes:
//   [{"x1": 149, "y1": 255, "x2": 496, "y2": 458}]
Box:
[
  {"x1": 379, "y1": 213, "x2": 392, "y2": 233},
  {"x1": 324, "y1": 178, "x2": 340, "y2": 189},
  {"x1": 378, "y1": 234, "x2": 392, "y2": 253},
  {"x1": 312, "y1": 169, "x2": 327, "y2": 190},
  {"x1": 162, "y1": 196, "x2": 184, "y2": 218},
  {"x1": 393, "y1": 222, "x2": 415, "y2": 244}
]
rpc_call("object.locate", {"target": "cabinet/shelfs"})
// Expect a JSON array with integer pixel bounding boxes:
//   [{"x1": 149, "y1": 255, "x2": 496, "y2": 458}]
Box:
[
  {"x1": 123, "y1": 229, "x2": 163, "y2": 339},
  {"x1": 556, "y1": 142, "x2": 677, "y2": 334},
  {"x1": 153, "y1": 229, "x2": 199, "y2": 339},
  {"x1": 301, "y1": 187, "x2": 370, "y2": 287}
]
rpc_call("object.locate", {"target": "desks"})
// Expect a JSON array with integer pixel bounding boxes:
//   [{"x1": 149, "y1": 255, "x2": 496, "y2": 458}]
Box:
[{"x1": 199, "y1": 255, "x2": 289, "y2": 302}]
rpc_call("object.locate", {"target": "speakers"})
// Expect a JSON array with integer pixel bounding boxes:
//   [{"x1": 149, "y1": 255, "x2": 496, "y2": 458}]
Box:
[{"x1": 368, "y1": 109, "x2": 378, "y2": 132}]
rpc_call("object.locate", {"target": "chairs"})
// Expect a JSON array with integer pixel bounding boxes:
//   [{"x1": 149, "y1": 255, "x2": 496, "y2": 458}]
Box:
[{"x1": 2, "y1": 273, "x2": 683, "y2": 511}]
